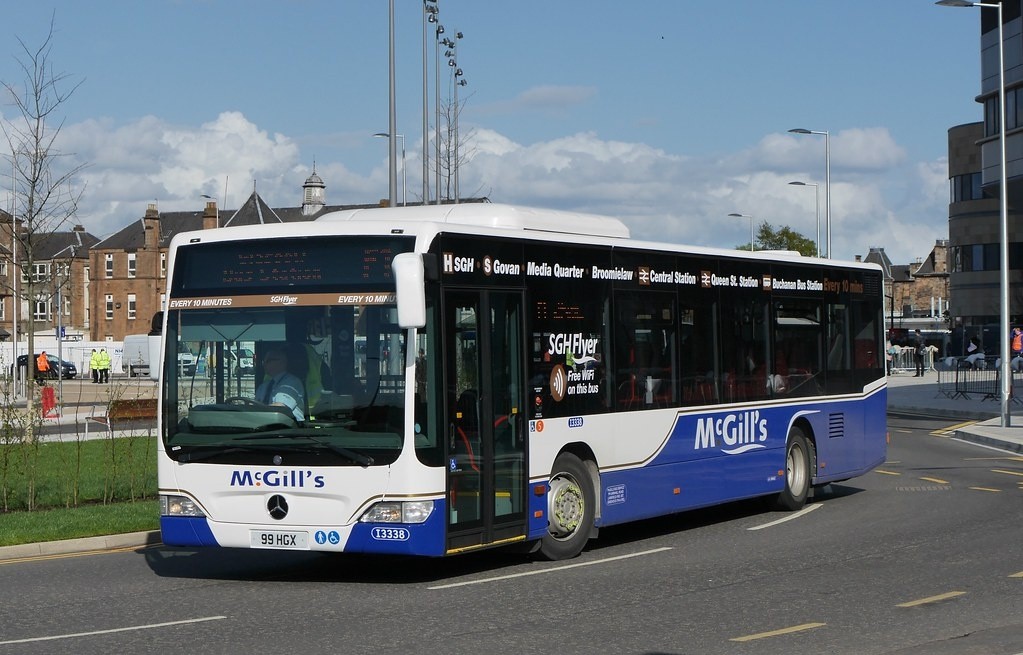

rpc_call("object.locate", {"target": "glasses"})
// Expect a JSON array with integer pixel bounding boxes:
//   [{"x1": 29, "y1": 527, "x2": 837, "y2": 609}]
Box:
[{"x1": 261, "y1": 358, "x2": 279, "y2": 367}]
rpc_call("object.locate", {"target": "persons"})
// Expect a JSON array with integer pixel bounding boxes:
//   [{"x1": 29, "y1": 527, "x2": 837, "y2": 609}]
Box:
[
  {"x1": 99, "y1": 349, "x2": 110, "y2": 383},
  {"x1": 253, "y1": 350, "x2": 305, "y2": 426},
  {"x1": 967, "y1": 337, "x2": 981, "y2": 371},
  {"x1": 1011, "y1": 327, "x2": 1023, "y2": 373},
  {"x1": 885, "y1": 336, "x2": 893, "y2": 376},
  {"x1": 913, "y1": 329, "x2": 925, "y2": 377},
  {"x1": 37, "y1": 351, "x2": 50, "y2": 385},
  {"x1": 90, "y1": 349, "x2": 100, "y2": 383}
]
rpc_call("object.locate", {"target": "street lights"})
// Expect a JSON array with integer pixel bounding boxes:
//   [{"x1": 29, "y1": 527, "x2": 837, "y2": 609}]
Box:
[
  {"x1": 788, "y1": 181, "x2": 820, "y2": 258},
  {"x1": 371, "y1": 133, "x2": 406, "y2": 208},
  {"x1": 934, "y1": 0, "x2": 1012, "y2": 426},
  {"x1": 728, "y1": 213, "x2": 754, "y2": 252},
  {"x1": 787, "y1": 128, "x2": 832, "y2": 260},
  {"x1": 200, "y1": 195, "x2": 218, "y2": 228},
  {"x1": 13, "y1": 150, "x2": 47, "y2": 402}
]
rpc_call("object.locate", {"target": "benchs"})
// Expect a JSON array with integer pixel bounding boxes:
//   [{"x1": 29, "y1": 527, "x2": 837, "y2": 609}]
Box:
[{"x1": 85, "y1": 399, "x2": 158, "y2": 441}]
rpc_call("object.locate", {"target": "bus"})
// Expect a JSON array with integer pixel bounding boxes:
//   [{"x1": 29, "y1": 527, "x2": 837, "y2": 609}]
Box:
[{"x1": 147, "y1": 200, "x2": 893, "y2": 563}]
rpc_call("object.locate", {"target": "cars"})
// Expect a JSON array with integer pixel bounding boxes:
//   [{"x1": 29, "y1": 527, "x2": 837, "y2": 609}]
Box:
[{"x1": 11, "y1": 354, "x2": 77, "y2": 380}]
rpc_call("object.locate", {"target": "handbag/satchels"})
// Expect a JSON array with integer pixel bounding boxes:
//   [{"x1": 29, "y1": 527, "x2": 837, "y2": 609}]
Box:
[{"x1": 888, "y1": 347, "x2": 896, "y2": 355}]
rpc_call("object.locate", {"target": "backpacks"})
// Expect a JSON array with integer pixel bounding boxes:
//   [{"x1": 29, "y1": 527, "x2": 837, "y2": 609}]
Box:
[{"x1": 916, "y1": 335, "x2": 927, "y2": 356}]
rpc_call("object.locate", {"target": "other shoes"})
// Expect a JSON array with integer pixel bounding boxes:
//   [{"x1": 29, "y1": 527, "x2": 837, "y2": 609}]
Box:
[
  {"x1": 887, "y1": 373, "x2": 891, "y2": 376},
  {"x1": 913, "y1": 374, "x2": 920, "y2": 377},
  {"x1": 921, "y1": 374, "x2": 924, "y2": 377}
]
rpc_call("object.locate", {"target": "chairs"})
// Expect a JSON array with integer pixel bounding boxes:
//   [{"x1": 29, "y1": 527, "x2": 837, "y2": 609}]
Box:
[{"x1": 599, "y1": 367, "x2": 811, "y2": 408}]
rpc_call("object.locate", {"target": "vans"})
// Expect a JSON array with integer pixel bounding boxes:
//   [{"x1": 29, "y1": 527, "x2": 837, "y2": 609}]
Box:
[
  {"x1": 121, "y1": 334, "x2": 196, "y2": 377},
  {"x1": 223, "y1": 347, "x2": 256, "y2": 376}
]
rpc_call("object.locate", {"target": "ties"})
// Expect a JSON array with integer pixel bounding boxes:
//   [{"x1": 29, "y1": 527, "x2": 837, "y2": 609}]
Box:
[{"x1": 264, "y1": 378, "x2": 275, "y2": 405}]
